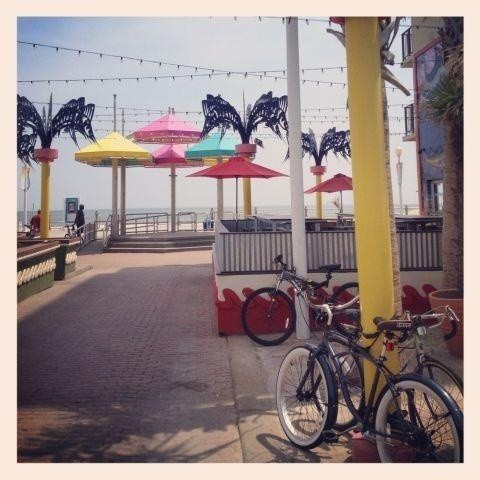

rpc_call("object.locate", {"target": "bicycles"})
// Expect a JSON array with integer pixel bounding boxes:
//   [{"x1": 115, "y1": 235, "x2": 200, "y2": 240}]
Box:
[
  {"x1": 64, "y1": 224, "x2": 84, "y2": 247},
  {"x1": 275, "y1": 278, "x2": 463, "y2": 463},
  {"x1": 24, "y1": 224, "x2": 40, "y2": 239},
  {"x1": 310, "y1": 304, "x2": 463, "y2": 463},
  {"x1": 241, "y1": 254, "x2": 361, "y2": 346}
]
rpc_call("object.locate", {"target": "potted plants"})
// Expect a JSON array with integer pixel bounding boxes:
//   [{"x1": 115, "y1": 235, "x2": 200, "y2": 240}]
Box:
[{"x1": 420, "y1": 16, "x2": 464, "y2": 359}]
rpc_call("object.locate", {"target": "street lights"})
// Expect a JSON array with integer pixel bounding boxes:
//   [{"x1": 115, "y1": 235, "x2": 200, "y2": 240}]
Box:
[{"x1": 395, "y1": 146, "x2": 404, "y2": 215}]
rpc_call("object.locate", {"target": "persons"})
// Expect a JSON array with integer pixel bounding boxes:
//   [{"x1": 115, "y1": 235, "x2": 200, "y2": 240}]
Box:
[
  {"x1": 74, "y1": 204, "x2": 85, "y2": 236},
  {"x1": 30, "y1": 208, "x2": 41, "y2": 232}
]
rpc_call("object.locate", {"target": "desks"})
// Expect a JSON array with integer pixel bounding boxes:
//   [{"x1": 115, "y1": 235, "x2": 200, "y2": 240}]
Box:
[{"x1": 243, "y1": 213, "x2": 443, "y2": 233}]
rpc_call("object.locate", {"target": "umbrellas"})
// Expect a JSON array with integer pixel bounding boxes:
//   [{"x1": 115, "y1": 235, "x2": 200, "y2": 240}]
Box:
[
  {"x1": 186, "y1": 155, "x2": 290, "y2": 219},
  {"x1": 304, "y1": 172, "x2": 353, "y2": 213}
]
